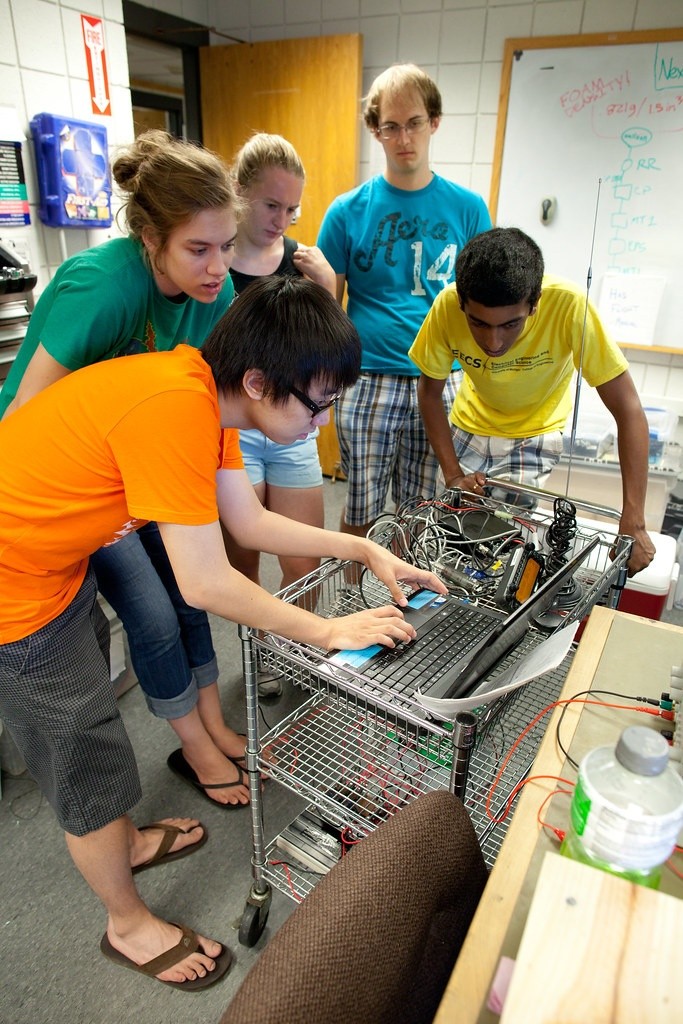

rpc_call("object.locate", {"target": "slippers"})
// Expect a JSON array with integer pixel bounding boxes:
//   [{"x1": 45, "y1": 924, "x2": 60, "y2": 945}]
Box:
[
  {"x1": 132, "y1": 818, "x2": 206, "y2": 874},
  {"x1": 100, "y1": 920, "x2": 232, "y2": 991},
  {"x1": 168, "y1": 732, "x2": 276, "y2": 810}
]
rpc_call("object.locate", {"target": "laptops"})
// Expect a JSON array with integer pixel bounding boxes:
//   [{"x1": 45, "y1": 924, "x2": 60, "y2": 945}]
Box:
[{"x1": 309, "y1": 536, "x2": 601, "y2": 736}]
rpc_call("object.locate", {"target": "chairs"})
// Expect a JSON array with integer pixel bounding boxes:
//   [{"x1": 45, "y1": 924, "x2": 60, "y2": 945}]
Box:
[{"x1": 213, "y1": 790, "x2": 490, "y2": 1023}]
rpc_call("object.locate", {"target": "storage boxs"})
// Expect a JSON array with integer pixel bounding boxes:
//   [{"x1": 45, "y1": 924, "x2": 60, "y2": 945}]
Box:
[{"x1": 508, "y1": 508, "x2": 681, "y2": 650}]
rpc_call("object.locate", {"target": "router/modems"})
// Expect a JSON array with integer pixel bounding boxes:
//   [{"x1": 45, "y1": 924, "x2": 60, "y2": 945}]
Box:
[{"x1": 438, "y1": 508, "x2": 522, "y2": 554}]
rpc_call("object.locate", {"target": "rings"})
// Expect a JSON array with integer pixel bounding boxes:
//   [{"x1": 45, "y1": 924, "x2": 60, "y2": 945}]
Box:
[{"x1": 472, "y1": 483, "x2": 479, "y2": 491}]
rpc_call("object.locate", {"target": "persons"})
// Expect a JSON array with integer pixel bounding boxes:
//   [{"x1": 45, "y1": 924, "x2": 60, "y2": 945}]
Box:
[
  {"x1": 0, "y1": 276, "x2": 450, "y2": 992},
  {"x1": 404, "y1": 226, "x2": 662, "y2": 580},
  {"x1": 229, "y1": 133, "x2": 337, "y2": 703},
  {"x1": 315, "y1": 62, "x2": 494, "y2": 620},
  {"x1": 2, "y1": 127, "x2": 279, "y2": 809}
]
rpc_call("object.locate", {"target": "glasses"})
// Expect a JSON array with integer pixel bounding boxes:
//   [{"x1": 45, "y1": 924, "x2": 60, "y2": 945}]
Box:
[
  {"x1": 287, "y1": 383, "x2": 346, "y2": 416},
  {"x1": 372, "y1": 116, "x2": 431, "y2": 139}
]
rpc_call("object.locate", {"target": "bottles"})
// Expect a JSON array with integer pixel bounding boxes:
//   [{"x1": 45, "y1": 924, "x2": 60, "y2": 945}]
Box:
[{"x1": 563, "y1": 724, "x2": 682, "y2": 893}]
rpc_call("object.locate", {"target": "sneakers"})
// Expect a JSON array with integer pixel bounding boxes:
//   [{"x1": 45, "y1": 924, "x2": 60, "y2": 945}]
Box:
[{"x1": 251, "y1": 655, "x2": 284, "y2": 697}]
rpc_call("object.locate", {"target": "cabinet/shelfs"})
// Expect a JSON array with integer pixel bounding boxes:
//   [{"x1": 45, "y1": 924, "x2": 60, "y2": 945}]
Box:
[{"x1": 432, "y1": 603, "x2": 678, "y2": 1024}]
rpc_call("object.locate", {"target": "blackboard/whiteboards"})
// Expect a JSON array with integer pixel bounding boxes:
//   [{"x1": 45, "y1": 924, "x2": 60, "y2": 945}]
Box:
[{"x1": 489, "y1": 27, "x2": 683, "y2": 355}]
